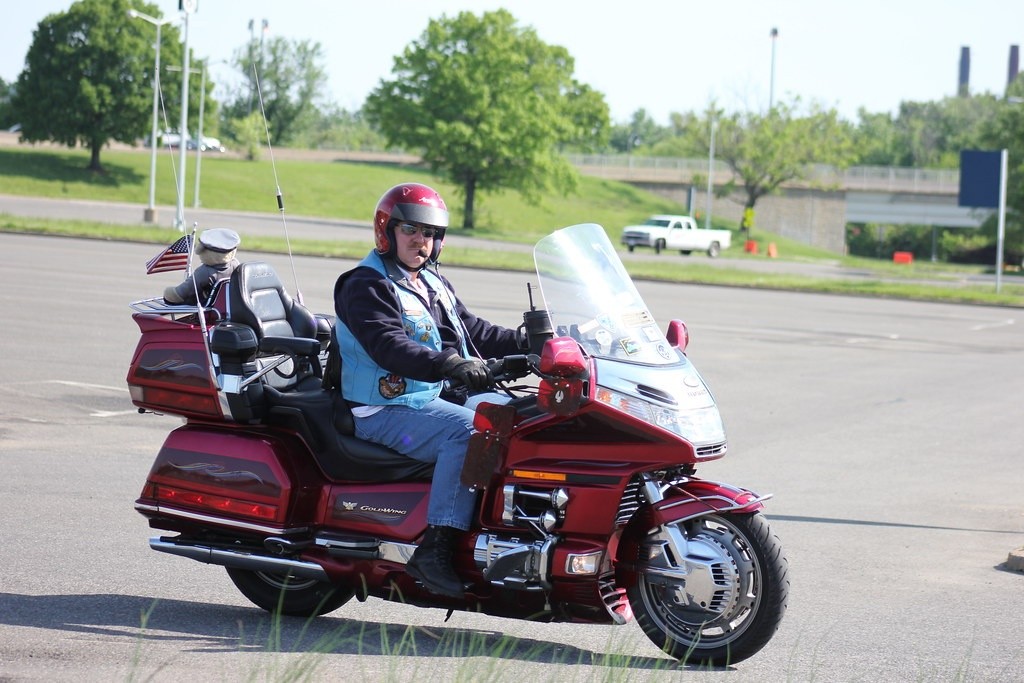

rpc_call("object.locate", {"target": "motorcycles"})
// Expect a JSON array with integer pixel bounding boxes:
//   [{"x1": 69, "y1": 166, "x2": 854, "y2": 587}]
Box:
[{"x1": 126, "y1": 221, "x2": 789, "y2": 671}]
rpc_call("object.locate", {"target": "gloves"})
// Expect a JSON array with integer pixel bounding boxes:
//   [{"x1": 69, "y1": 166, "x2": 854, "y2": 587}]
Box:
[{"x1": 442, "y1": 353, "x2": 494, "y2": 391}]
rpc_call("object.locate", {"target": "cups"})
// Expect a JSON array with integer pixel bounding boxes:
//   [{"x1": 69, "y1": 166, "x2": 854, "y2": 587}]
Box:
[{"x1": 515, "y1": 310, "x2": 555, "y2": 355}]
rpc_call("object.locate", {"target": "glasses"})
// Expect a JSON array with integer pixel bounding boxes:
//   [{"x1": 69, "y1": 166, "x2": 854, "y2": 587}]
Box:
[{"x1": 395, "y1": 223, "x2": 444, "y2": 237}]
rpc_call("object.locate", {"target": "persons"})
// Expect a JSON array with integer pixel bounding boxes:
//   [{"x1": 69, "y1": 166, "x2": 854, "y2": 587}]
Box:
[{"x1": 334, "y1": 184, "x2": 528, "y2": 597}]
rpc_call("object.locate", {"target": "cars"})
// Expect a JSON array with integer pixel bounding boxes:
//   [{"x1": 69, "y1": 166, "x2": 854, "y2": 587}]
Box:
[{"x1": 147, "y1": 127, "x2": 221, "y2": 151}]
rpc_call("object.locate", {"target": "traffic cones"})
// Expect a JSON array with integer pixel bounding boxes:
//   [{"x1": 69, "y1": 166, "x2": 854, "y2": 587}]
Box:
[{"x1": 765, "y1": 241, "x2": 777, "y2": 258}]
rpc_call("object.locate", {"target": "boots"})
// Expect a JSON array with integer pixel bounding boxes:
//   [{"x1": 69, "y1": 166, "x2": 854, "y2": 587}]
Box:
[{"x1": 403, "y1": 525, "x2": 464, "y2": 598}]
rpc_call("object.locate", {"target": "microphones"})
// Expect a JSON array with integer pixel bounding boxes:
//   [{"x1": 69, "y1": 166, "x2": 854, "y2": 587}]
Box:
[{"x1": 418, "y1": 248, "x2": 437, "y2": 265}]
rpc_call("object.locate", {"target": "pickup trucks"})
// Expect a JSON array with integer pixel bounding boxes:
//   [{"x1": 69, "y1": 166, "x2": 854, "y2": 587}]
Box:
[{"x1": 622, "y1": 214, "x2": 732, "y2": 259}]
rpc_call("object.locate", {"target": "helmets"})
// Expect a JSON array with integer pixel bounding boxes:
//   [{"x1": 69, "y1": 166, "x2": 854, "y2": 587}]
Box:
[{"x1": 374, "y1": 183, "x2": 448, "y2": 265}]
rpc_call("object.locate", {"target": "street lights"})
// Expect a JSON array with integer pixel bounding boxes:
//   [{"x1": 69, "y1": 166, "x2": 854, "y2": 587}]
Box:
[
  {"x1": 770, "y1": 27, "x2": 779, "y2": 111},
  {"x1": 193, "y1": 57, "x2": 230, "y2": 208},
  {"x1": 127, "y1": 6, "x2": 187, "y2": 224},
  {"x1": 705, "y1": 119, "x2": 719, "y2": 229}
]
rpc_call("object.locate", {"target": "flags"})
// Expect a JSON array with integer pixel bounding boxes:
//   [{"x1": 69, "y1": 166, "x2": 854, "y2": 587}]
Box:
[{"x1": 146, "y1": 233, "x2": 195, "y2": 275}]
